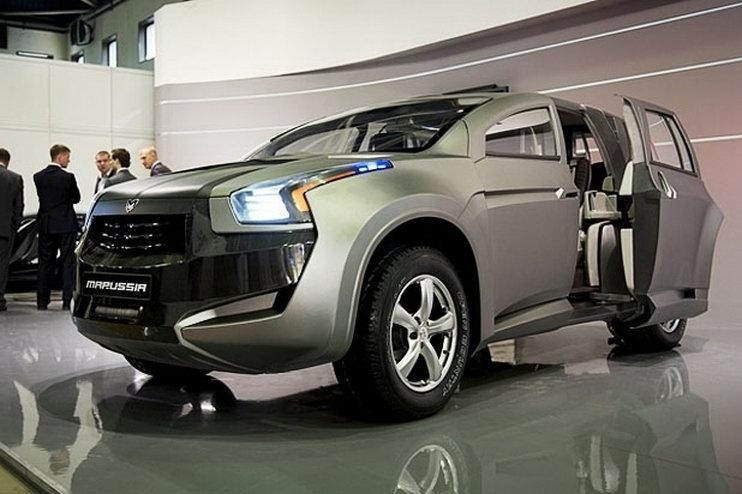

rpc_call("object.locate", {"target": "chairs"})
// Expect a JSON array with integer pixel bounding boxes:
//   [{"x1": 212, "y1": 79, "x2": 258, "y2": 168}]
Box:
[
  {"x1": 368, "y1": 132, "x2": 408, "y2": 151},
  {"x1": 567, "y1": 157, "x2": 592, "y2": 251}
]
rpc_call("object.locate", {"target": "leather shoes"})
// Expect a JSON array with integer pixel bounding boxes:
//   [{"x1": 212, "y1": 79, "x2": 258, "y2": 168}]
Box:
[
  {"x1": 38, "y1": 305, "x2": 47, "y2": 310},
  {"x1": 63, "y1": 303, "x2": 69, "y2": 309}
]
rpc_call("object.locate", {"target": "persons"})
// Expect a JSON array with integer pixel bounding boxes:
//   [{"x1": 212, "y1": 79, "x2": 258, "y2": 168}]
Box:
[
  {"x1": 0, "y1": 148, "x2": 24, "y2": 312},
  {"x1": 105, "y1": 149, "x2": 136, "y2": 190},
  {"x1": 33, "y1": 144, "x2": 81, "y2": 311},
  {"x1": 140, "y1": 147, "x2": 170, "y2": 176},
  {"x1": 93, "y1": 151, "x2": 114, "y2": 196}
]
